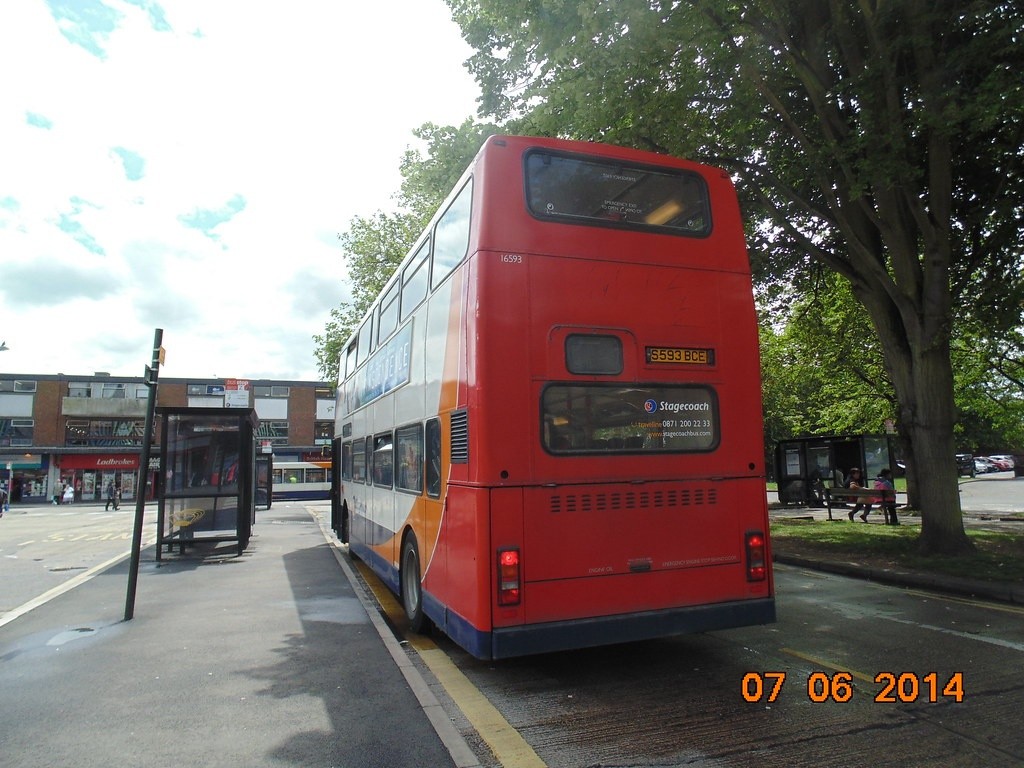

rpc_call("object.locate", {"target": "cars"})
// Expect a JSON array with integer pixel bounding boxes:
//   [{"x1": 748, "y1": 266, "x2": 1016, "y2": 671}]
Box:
[
  {"x1": 956, "y1": 454, "x2": 975, "y2": 479},
  {"x1": 973, "y1": 455, "x2": 1014, "y2": 473},
  {"x1": 975, "y1": 461, "x2": 989, "y2": 474}
]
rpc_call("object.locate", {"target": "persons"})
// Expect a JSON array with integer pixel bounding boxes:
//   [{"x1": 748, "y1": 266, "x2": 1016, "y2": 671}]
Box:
[
  {"x1": 53, "y1": 479, "x2": 62, "y2": 505},
  {"x1": 810, "y1": 465, "x2": 824, "y2": 492},
  {"x1": 63, "y1": 484, "x2": 75, "y2": 504},
  {"x1": 844, "y1": 468, "x2": 872, "y2": 523},
  {"x1": 290, "y1": 474, "x2": 296, "y2": 483},
  {"x1": 874, "y1": 468, "x2": 900, "y2": 525},
  {"x1": 0, "y1": 488, "x2": 7, "y2": 518},
  {"x1": 828, "y1": 465, "x2": 843, "y2": 487},
  {"x1": 104, "y1": 479, "x2": 122, "y2": 511}
]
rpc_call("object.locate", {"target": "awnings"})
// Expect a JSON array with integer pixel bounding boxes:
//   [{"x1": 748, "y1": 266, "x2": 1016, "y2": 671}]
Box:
[
  {"x1": 60, "y1": 454, "x2": 139, "y2": 469},
  {"x1": 0, "y1": 455, "x2": 42, "y2": 469}
]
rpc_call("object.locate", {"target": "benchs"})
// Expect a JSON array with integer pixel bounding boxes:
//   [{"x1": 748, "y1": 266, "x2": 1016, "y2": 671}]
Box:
[
  {"x1": 825, "y1": 488, "x2": 901, "y2": 525},
  {"x1": 169, "y1": 510, "x2": 205, "y2": 555}
]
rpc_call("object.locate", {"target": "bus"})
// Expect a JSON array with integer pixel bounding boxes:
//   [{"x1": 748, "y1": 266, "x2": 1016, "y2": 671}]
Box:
[
  {"x1": 330, "y1": 133, "x2": 779, "y2": 661},
  {"x1": 255, "y1": 445, "x2": 332, "y2": 500}
]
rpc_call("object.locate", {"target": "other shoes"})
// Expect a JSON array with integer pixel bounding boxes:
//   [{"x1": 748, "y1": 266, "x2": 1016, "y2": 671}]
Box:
[
  {"x1": 105, "y1": 505, "x2": 108, "y2": 510},
  {"x1": 860, "y1": 514, "x2": 868, "y2": 523},
  {"x1": 115, "y1": 508, "x2": 120, "y2": 511},
  {"x1": 848, "y1": 512, "x2": 854, "y2": 522}
]
